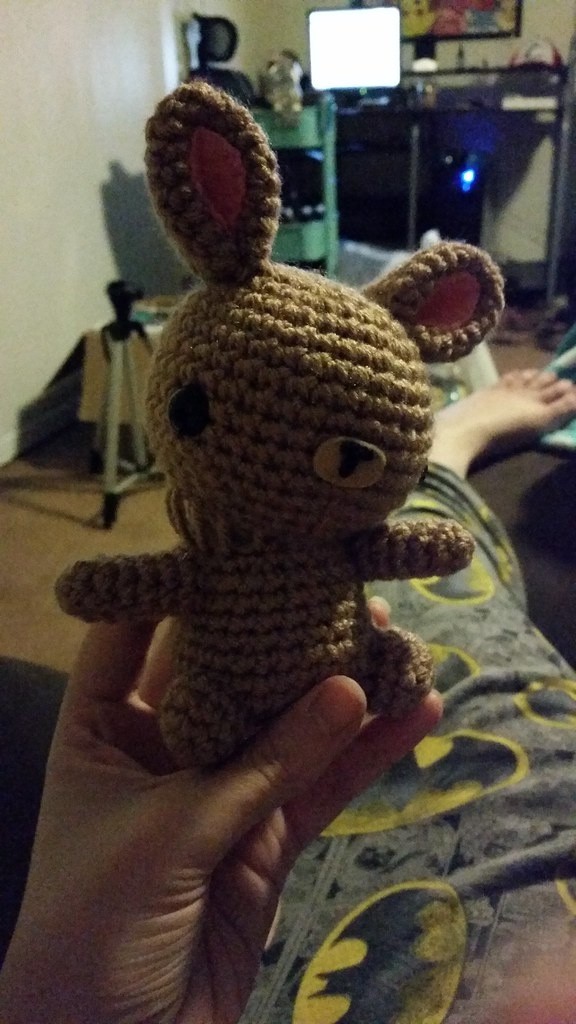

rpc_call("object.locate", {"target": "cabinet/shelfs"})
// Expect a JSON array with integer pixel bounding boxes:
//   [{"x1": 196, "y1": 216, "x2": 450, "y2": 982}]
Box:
[{"x1": 252, "y1": 92, "x2": 340, "y2": 281}]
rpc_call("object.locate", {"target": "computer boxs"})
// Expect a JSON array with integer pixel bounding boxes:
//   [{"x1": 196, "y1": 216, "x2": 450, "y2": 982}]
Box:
[{"x1": 424, "y1": 161, "x2": 484, "y2": 248}]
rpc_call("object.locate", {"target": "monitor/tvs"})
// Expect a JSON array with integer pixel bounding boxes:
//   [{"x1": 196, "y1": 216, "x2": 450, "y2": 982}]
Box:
[{"x1": 309, "y1": 6, "x2": 401, "y2": 90}]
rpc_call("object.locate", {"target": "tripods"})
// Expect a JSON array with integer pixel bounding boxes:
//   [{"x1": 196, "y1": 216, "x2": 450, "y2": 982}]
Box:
[{"x1": 88, "y1": 280, "x2": 161, "y2": 529}]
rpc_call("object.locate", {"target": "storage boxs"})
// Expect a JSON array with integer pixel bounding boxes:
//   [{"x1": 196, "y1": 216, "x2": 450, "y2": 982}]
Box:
[{"x1": 40, "y1": 329, "x2": 163, "y2": 475}]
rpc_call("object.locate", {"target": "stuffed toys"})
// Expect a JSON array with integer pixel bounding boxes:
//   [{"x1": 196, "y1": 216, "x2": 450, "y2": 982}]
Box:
[{"x1": 52, "y1": 84, "x2": 508, "y2": 775}]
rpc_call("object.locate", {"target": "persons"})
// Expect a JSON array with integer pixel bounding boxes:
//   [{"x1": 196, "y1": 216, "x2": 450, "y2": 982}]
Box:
[{"x1": 0, "y1": 368, "x2": 576, "y2": 1024}]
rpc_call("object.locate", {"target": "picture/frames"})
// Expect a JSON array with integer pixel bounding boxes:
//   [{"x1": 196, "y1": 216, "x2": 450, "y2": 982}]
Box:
[{"x1": 350, "y1": 0, "x2": 522, "y2": 42}]
